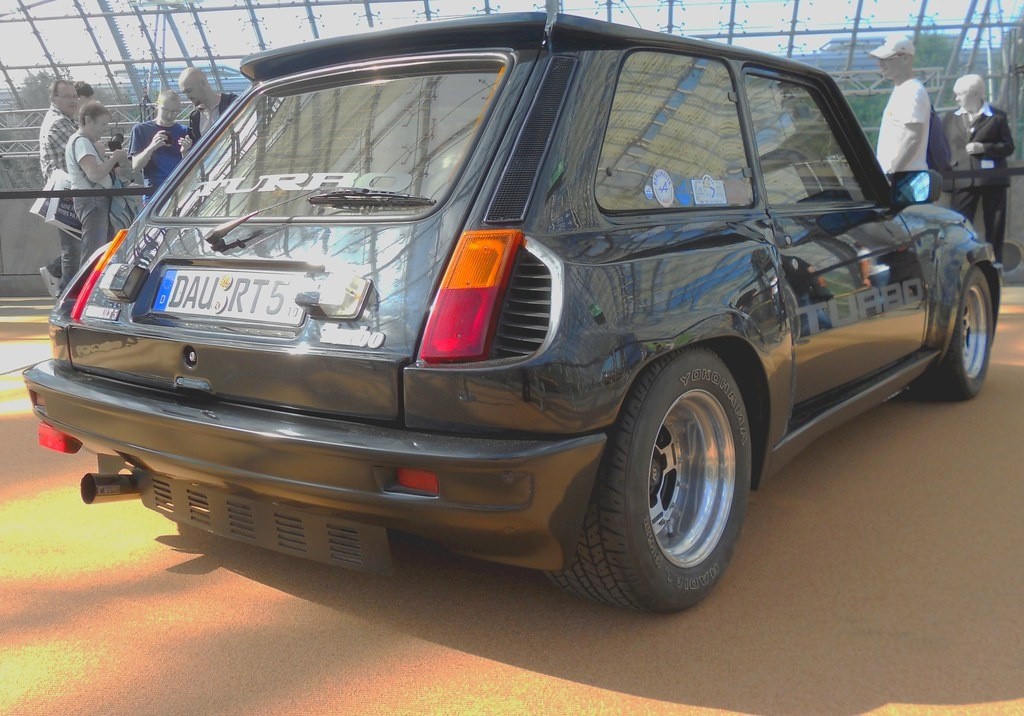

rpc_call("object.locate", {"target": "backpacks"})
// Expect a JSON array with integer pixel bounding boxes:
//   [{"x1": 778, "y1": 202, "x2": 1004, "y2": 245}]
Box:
[{"x1": 926, "y1": 103, "x2": 952, "y2": 172}]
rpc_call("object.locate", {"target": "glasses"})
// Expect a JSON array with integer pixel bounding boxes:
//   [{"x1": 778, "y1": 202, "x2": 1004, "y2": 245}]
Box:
[
  {"x1": 54, "y1": 93, "x2": 80, "y2": 101},
  {"x1": 876, "y1": 54, "x2": 906, "y2": 66},
  {"x1": 158, "y1": 107, "x2": 180, "y2": 115}
]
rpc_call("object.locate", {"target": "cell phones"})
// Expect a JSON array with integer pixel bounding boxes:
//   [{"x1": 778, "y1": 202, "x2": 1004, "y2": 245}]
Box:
[{"x1": 163, "y1": 132, "x2": 173, "y2": 145}]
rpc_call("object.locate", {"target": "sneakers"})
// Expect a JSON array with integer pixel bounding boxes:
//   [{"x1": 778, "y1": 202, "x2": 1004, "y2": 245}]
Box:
[{"x1": 40, "y1": 267, "x2": 62, "y2": 301}]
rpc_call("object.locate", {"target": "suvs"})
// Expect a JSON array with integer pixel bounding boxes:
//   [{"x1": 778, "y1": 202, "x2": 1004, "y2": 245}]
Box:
[{"x1": 21, "y1": 10, "x2": 1002, "y2": 613}]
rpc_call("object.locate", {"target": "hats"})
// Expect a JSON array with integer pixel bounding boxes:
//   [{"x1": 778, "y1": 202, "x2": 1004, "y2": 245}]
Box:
[{"x1": 869, "y1": 34, "x2": 915, "y2": 59}]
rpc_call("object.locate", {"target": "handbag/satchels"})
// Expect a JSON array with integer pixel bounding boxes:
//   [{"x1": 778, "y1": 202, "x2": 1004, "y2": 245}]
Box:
[
  {"x1": 29, "y1": 169, "x2": 83, "y2": 241},
  {"x1": 110, "y1": 175, "x2": 139, "y2": 233}
]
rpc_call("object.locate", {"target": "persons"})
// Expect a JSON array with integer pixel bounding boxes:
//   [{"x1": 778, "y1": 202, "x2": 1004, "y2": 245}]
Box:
[
  {"x1": 853, "y1": 241, "x2": 879, "y2": 288},
  {"x1": 942, "y1": 75, "x2": 1015, "y2": 263},
  {"x1": 37, "y1": 68, "x2": 239, "y2": 299},
  {"x1": 751, "y1": 245, "x2": 835, "y2": 341},
  {"x1": 869, "y1": 34, "x2": 932, "y2": 187}
]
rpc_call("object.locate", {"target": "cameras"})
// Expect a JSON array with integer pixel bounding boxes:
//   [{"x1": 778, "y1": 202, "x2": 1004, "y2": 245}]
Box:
[{"x1": 108, "y1": 133, "x2": 125, "y2": 150}]
